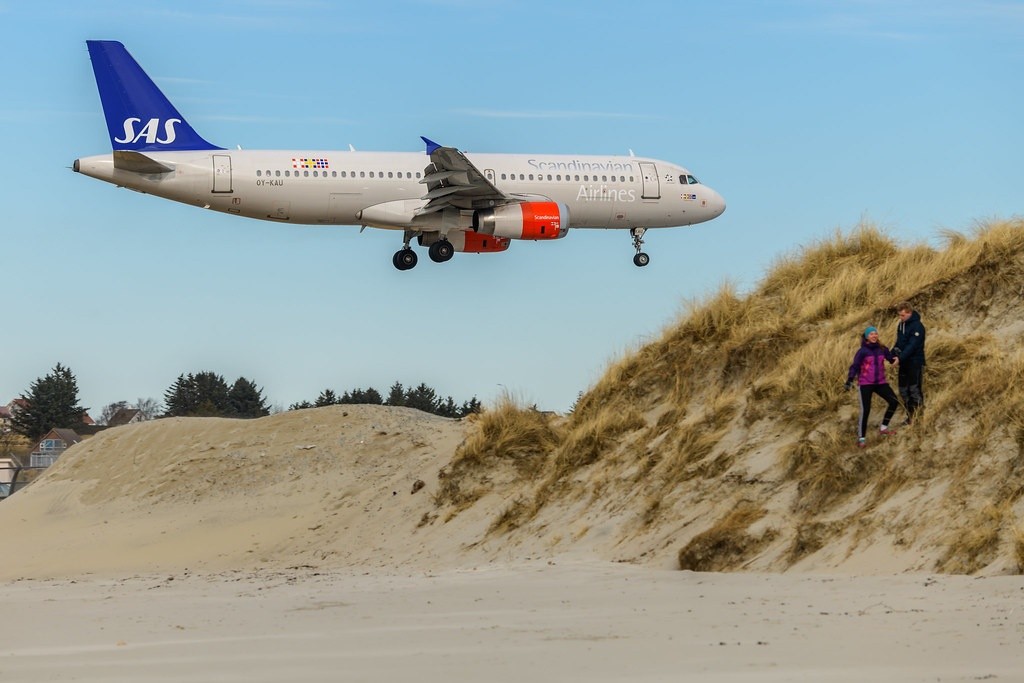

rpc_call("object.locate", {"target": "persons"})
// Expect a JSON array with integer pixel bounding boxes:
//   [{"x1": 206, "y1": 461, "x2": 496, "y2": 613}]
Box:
[
  {"x1": 889, "y1": 303, "x2": 926, "y2": 426},
  {"x1": 844, "y1": 327, "x2": 899, "y2": 447}
]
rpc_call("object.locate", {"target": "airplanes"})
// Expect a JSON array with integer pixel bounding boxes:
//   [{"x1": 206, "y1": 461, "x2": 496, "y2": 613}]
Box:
[{"x1": 72, "y1": 37, "x2": 727, "y2": 271}]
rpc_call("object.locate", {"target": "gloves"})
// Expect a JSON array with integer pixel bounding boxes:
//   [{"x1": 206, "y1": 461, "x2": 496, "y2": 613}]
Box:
[
  {"x1": 890, "y1": 347, "x2": 902, "y2": 357},
  {"x1": 844, "y1": 377, "x2": 855, "y2": 391}
]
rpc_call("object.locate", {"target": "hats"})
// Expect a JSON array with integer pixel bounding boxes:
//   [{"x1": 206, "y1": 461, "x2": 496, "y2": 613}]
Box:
[{"x1": 864, "y1": 326, "x2": 877, "y2": 339}]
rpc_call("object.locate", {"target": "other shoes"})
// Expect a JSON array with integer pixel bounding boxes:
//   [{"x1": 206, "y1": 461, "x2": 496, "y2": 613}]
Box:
[
  {"x1": 880, "y1": 428, "x2": 897, "y2": 436},
  {"x1": 856, "y1": 439, "x2": 866, "y2": 449},
  {"x1": 901, "y1": 418, "x2": 911, "y2": 426}
]
